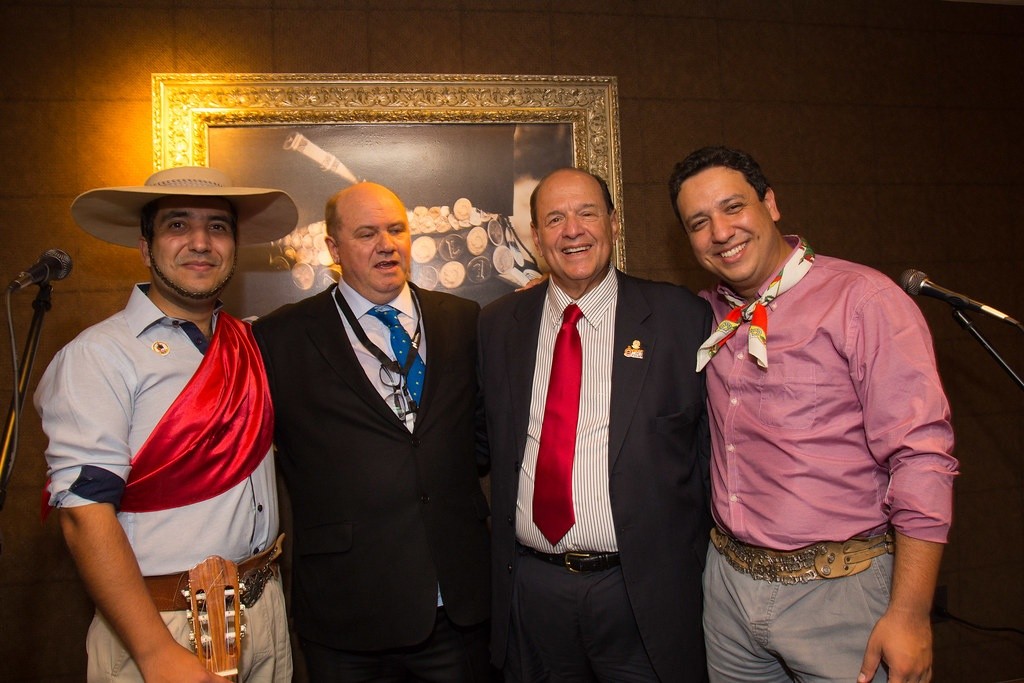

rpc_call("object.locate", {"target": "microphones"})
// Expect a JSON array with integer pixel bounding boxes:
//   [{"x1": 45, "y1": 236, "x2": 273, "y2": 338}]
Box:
[
  {"x1": 7, "y1": 249, "x2": 72, "y2": 293},
  {"x1": 900, "y1": 269, "x2": 1020, "y2": 327}
]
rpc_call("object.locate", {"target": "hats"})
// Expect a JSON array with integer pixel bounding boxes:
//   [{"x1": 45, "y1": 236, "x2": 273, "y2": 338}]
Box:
[{"x1": 70, "y1": 167, "x2": 299, "y2": 248}]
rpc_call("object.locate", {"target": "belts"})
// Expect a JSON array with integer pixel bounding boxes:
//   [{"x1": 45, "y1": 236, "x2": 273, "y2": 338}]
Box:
[
  {"x1": 140, "y1": 533, "x2": 285, "y2": 611},
  {"x1": 515, "y1": 541, "x2": 620, "y2": 574},
  {"x1": 710, "y1": 524, "x2": 896, "y2": 585}
]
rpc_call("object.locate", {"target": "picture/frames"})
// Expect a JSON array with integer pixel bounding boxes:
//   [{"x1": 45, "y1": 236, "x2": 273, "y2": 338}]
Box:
[{"x1": 147, "y1": 68, "x2": 629, "y2": 326}]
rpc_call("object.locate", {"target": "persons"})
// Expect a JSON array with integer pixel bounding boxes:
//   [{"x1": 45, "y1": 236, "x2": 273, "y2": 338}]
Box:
[
  {"x1": 478, "y1": 166, "x2": 715, "y2": 683},
  {"x1": 251, "y1": 182, "x2": 482, "y2": 683},
  {"x1": 514, "y1": 145, "x2": 961, "y2": 683},
  {"x1": 32, "y1": 166, "x2": 299, "y2": 683}
]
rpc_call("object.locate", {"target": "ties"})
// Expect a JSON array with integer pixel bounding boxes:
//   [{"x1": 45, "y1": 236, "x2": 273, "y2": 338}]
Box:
[
  {"x1": 366, "y1": 304, "x2": 426, "y2": 407},
  {"x1": 696, "y1": 236, "x2": 816, "y2": 373},
  {"x1": 532, "y1": 304, "x2": 584, "y2": 546}
]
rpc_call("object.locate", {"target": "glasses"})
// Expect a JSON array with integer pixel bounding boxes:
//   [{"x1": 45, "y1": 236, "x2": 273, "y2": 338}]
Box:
[{"x1": 379, "y1": 360, "x2": 406, "y2": 424}]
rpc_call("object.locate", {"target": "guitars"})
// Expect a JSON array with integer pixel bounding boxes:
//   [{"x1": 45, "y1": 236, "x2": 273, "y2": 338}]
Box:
[{"x1": 180, "y1": 555, "x2": 249, "y2": 683}]
